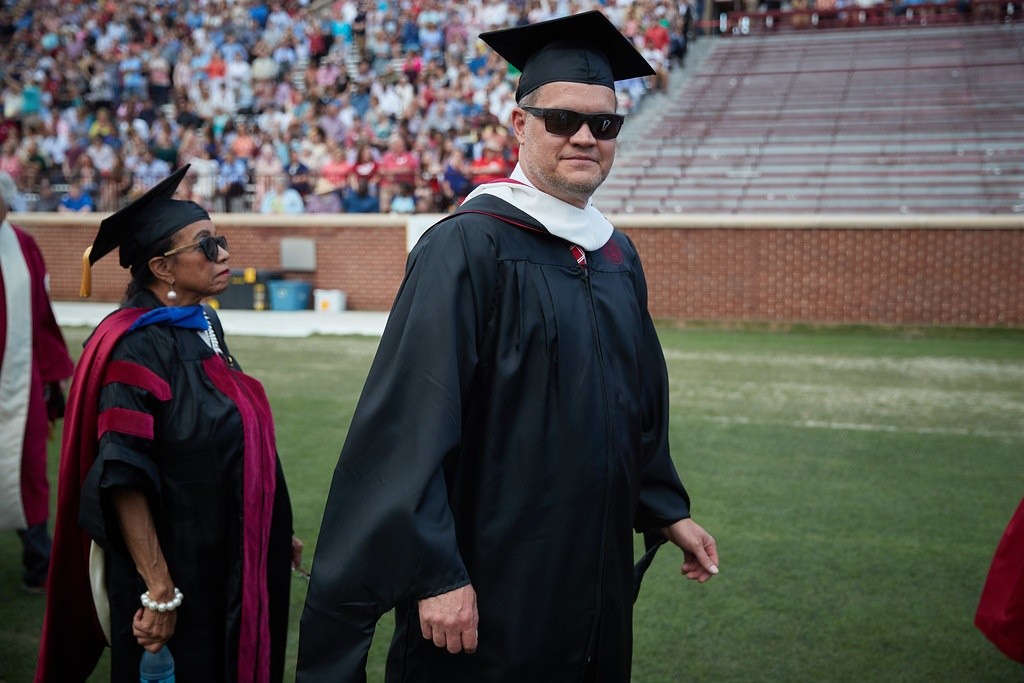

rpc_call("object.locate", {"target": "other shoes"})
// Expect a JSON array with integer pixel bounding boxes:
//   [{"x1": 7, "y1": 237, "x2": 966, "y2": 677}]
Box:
[{"x1": 21, "y1": 574, "x2": 45, "y2": 593}]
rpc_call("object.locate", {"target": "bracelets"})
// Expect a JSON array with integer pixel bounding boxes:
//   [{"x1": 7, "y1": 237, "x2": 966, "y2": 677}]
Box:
[{"x1": 138, "y1": 588, "x2": 183, "y2": 614}]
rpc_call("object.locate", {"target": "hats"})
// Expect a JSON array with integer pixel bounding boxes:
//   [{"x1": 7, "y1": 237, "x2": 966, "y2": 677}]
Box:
[
  {"x1": 78, "y1": 164, "x2": 210, "y2": 298},
  {"x1": 479, "y1": 9, "x2": 657, "y2": 103}
]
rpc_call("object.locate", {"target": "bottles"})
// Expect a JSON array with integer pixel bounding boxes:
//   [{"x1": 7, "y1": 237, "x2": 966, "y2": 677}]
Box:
[{"x1": 140, "y1": 644, "x2": 175, "y2": 683}]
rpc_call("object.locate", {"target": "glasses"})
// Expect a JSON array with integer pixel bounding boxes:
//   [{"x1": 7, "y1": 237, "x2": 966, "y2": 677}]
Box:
[
  {"x1": 521, "y1": 106, "x2": 625, "y2": 140},
  {"x1": 159, "y1": 235, "x2": 230, "y2": 261}
]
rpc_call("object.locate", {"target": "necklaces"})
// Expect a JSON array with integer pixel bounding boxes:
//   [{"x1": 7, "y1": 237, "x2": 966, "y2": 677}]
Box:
[{"x1": 200, "y1": 309, "x2": 221, "y2": 353}]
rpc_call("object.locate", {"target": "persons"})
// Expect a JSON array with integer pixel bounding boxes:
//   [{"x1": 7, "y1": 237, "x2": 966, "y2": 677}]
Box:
[
  {"x1": 0, "y1": 0, "x2": 1024, "y2": 216},
  {"x1": 34, "y1": 162, "x2": 303, "y2": 683},
  {"x1": 298, "y1": 11, "x2": 719, "y2": 683},
  {"x1": 0, "y1": 170, "x2": 75, "y2": 592}
]
